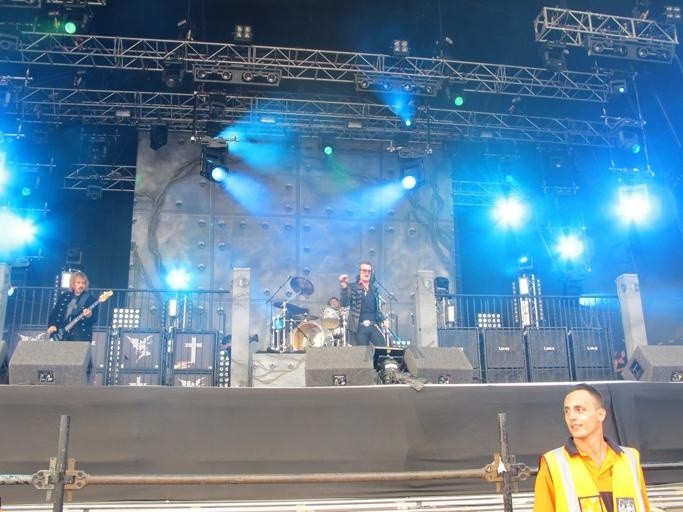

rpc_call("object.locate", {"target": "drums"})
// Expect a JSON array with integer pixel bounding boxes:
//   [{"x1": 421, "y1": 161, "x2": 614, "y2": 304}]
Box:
[
  {"x1": 291, "y1": 319, "x2": 334, "y2": 353},
  {"x1": 321, "y1": 305, "x2": 340, "y2": 330}
]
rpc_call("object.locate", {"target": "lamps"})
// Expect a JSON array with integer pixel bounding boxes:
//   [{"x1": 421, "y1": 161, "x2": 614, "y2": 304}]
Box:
[
  {"x1": 399, "y1": 158, "x2": 426, "y2": 192},
  {"x1": 354, "y1": 73, "x2": 438, "y2": 98},
  {"x1": 200, "y1": 148, "x2": 229, "y2": 183},
  {"x1": 616, "y1": 130, "x2": 641, "y2": 155},
  {"x1": 666, "y1": 5, "x2": 681, "y2": 20},
  {"x1": 150, "y1": 126, "x2": 168, "y2": 151},
  {"x1": 64, "y1": 12, "x2": 83, "y2": 34},
  {"x1": 192, "y1": 64, "x2": 281, "y2": 88},
  {"x1": 610, "y1": 79, "x2": 628, "y2": 95},
  {"x1": 391, "y1": 39, "x2": 410, "y2": 56},
  {"x1": 446, "y1": 86, "x2": 466, "y2": 107},
  {"x1": 317, "y1": 132, "x2": 337, "y2": 156},
  {"x1": 233, "y1": 22, "x2": 253, "y2": 45}
]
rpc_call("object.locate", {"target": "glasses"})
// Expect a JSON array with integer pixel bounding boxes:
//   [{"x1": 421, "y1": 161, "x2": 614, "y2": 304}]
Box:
[{"x1": 359, "y1": 269, "x2": 371, "y2": 274}]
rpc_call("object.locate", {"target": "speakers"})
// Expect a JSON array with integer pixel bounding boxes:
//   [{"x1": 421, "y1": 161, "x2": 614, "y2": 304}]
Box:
[
  {"x1": 405, "y1": 347, "x2": 473, "y2": 385},
  {"x1": 305, "y1": 346, "x2": 374, "y2": 386},
  {"x1": 622, "y1": 345, "x2": 683, "y2": 381},
  {"x1": 9, "y1": 340, "x2": 95, "y2": 386}
]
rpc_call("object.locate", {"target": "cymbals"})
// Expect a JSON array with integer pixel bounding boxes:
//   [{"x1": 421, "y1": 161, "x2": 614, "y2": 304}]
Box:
[
  {"x1": 290, "y1": 277, "x2": 315, "y2": 296},
  {"x1": 273, "y1": 302, "x2": 298, "y2": 310},
  {"x1": 293, "y1": 313, "x2": 318, "y2": 321}
]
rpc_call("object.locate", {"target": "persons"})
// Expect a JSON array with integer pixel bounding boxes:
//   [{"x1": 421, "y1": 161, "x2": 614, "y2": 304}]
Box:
[
  {"x1": 322, "y1": 296, "x2": 343, "y2": 348},
  {"x1": 533, "y1": 383, "x2": 651, "y2": 512},
  {"x1": 613, "y1": 349, "x2": 628, "y2": 381},
  {"x1": 47, "y1": 270, "x2": 99, "y2": 381},
  {"x1": 339, "y1": 261, "x2": 389, "y2": 347}
]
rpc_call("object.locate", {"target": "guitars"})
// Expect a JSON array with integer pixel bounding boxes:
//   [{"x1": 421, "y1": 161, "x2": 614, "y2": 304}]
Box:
[{"x1": 50, "y1": 290, "x2": 113, "y2": 341}]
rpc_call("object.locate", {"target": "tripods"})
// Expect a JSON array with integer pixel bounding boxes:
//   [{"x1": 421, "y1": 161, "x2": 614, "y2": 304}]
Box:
[
  {"x1": 272, "y1": 290, "x2": 298, "y2": 353},
  {"x1": 256, "y1": 277, "x2": 291, "y2": 353}
]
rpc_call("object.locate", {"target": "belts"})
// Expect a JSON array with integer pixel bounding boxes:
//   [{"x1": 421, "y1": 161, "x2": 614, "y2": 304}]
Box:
[{"x1": 360, "y1": 320, "x2": 377, "y2": 327}]
